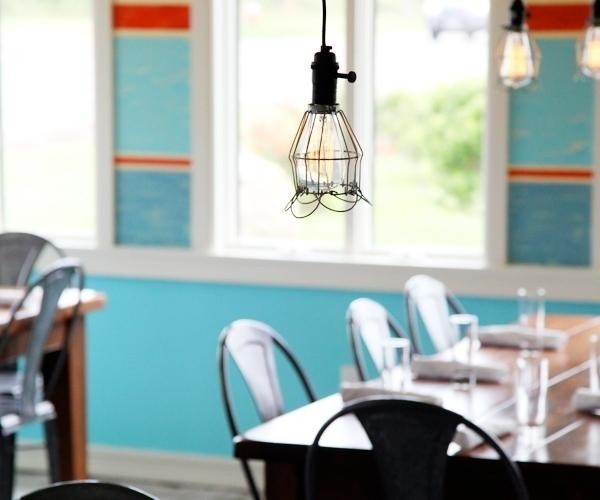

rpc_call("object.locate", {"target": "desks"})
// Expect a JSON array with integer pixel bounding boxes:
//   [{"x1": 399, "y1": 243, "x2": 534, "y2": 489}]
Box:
[
  {"x1": 2, "y1": 280, "x2": 106, "y2": 482},
  {"x1": 232, "y1": 314, "x2": 599, "y2": 500}
]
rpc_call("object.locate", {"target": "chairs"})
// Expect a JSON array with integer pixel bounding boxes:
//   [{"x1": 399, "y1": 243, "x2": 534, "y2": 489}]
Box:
[
  {"x1": 1, "y1": 233, "x2": 86, "y2": 376},
  {"x1": 1, "y1": 257, "x2": 86, "y2": 497},
  {"x1": 300, "y1": 394, "x2": 528, "y2": 499},
  {"x1": 401, "y1": 272, "x2": 475, "y2": 353},
  {"x1": 215, "y1": 317, "x2": 318, "y2": 497},
  {"x1": 17, "y1": 479, "x2": 158, "y2": 500},
  {"x1": 341, "y1": 296, "x2": 417, "y2": 383}
]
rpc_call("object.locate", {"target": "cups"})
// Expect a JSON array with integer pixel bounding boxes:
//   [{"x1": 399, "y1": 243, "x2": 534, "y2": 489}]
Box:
[
  {"x1": 449, "y1": 313, "x2": 480, "y2": 392},
  {"x1": 517, "y1": 286, "x2": 546, "y2": 352},
  {"x1": 516, "y1": 352, "x2": 549, "y2": 427},
  {"x1": 379, "y1": 337, "x2": 413, "y2": 395}
]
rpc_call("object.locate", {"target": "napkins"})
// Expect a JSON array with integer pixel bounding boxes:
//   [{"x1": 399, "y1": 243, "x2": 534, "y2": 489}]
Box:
[
  {"x1": 345, "y1": 373, "x2": 462, "y2": 406},
  {"x1": 569, "y1": 387, "x2": 600, "y2": 414},
  {"x1": 476, "y1": 322, "x2": 566, "y2": 352},
  {"x1": 434, "y1": 402, "x2": 514, "y2": 454},
  {"x1": 412, "y1": 347, "x2": 507, "y2": 386}
]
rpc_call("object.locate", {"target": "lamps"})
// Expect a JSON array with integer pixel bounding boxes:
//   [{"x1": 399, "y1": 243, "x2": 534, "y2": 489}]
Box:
[
  {"x1": 579, "y1": 1, "x2": 600, "y2": 80},
  {"x1": 284, "y1": 0, "x2": 374, "y2": 218},
  {"x1": 498, "y1": 0, "x2": 535, "y2": 91}
]
